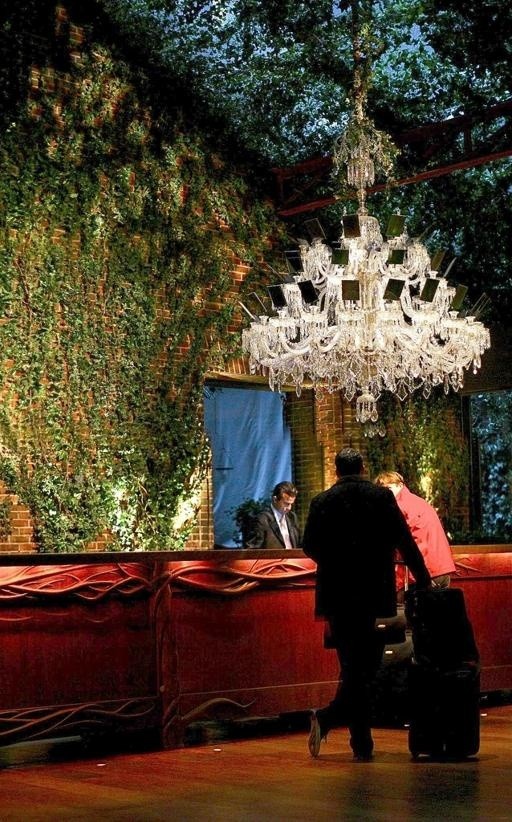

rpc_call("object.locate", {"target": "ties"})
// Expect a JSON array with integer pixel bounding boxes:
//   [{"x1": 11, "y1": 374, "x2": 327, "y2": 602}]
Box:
[{"x1": 280, "y1": 514, "x2": 292, "y2": 549}]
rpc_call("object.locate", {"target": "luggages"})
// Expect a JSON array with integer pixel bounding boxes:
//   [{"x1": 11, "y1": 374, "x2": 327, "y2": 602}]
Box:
[
  {"x1": 370, "y1": 560, "x2": 414, "y2": 731},
  {"x1": 408, "y1": 663, "x2": 481, "y2": 759}
]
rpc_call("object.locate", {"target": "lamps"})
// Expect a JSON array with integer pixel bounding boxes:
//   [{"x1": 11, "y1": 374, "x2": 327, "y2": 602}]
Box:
[{"x1": 238, "y1": 1, "x2": 491, "y2": 425}]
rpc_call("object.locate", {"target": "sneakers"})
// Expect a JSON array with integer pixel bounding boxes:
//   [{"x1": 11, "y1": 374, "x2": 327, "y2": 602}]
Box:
[{"x1": 308, "y1": 709, "x2": 323, "y2": 757}]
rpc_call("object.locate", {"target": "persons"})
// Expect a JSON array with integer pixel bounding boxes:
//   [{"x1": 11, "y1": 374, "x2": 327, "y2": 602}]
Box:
[
  {"x1": 294, "y1": 447, "x2": 436, "y2": 759},
  {"x1": 370, "y1": 471, "x2": 457, "y2": 648},
  {"x1": 242, "y1": 480, "x2": 301, "y2": 549}
]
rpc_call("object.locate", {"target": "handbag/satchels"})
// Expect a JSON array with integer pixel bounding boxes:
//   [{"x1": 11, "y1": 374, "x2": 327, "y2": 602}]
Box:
[{"x1": 412, "y1": 587, "x2": 466, "y2": 667}]
